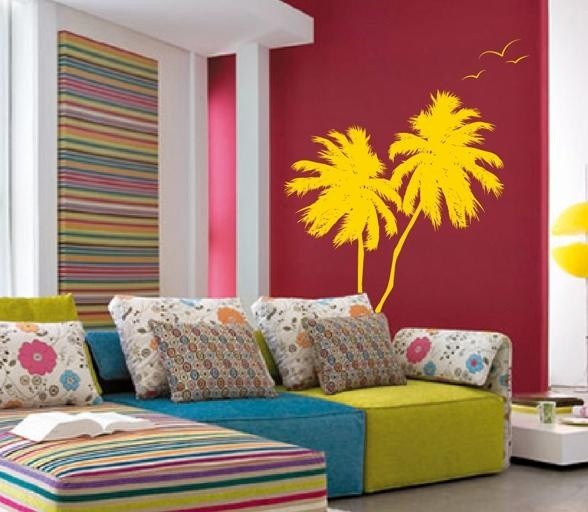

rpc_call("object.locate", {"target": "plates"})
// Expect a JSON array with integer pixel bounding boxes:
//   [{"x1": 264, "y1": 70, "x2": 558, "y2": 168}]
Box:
[{"x1": 560, "y1": 417, "x2": 588, "y2": 426}]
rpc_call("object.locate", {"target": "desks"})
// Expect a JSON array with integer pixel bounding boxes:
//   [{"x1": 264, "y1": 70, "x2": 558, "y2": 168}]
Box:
[{"x1": 509, "y1": 414, "x2": 588, "y2": 468}]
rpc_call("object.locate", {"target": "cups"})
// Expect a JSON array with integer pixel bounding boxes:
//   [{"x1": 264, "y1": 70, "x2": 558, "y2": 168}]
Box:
[{"x1": 537, "y1": 401, "x2": 556, "y2": 427}]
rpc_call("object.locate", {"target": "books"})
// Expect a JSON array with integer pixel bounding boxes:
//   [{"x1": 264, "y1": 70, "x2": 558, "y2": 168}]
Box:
[{"x1": 7, "y1": 408, "x2": 157, "y2": 443}]
